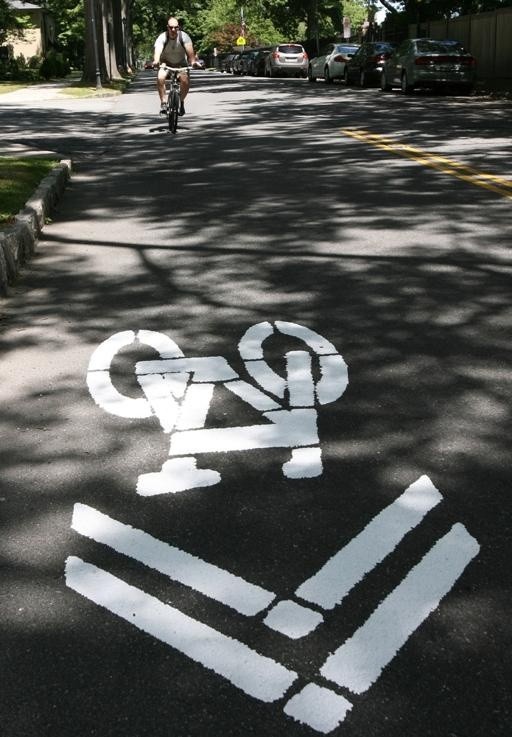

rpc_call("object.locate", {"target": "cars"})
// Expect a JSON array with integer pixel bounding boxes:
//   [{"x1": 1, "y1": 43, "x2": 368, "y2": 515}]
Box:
[
  {"x1": 221, "y1": 38, "x2": 477, "y2": 94},
  {"x1": 199, "y1": 60, "x2": 205, "y2": 70},
  {"x1": 144, "y1": 61, "x2": 154, "y2": 70}
]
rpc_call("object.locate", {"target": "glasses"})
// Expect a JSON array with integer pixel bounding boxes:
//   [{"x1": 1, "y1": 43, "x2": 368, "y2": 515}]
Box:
[{"x1": 169, "y1": 26, "x2": 179, "y2": 30}]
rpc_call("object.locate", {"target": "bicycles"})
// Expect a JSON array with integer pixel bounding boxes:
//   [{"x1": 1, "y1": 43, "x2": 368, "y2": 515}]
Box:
[{"x1": 154, "y1": 62, "x2": 205, "y2": 134}]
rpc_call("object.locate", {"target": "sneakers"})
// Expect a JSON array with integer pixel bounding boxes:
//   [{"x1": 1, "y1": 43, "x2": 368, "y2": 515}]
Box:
[
  {"x1": 178, "y1": 102, "x2": 185, "y2": 116},
  {"x1": 161, "y1": 102, "x2": 169, "y2": 113}
]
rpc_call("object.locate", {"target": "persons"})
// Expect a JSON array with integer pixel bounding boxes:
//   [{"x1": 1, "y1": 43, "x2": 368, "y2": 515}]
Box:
[{"x1": 151, "y1": 16, "x2": 203, "y2": 116}]
